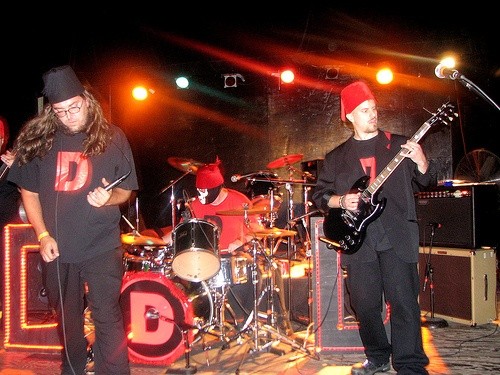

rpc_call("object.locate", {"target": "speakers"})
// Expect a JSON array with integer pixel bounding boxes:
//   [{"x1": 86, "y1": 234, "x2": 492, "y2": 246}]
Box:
[{"x1": 414, "y1": 186, "x2": 500, "y2": 326}]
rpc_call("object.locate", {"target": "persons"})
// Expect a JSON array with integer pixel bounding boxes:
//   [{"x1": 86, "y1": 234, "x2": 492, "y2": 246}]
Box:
[
  {"x1": 160, "y1": 163, "x2": 264, "y2": 333},
  {"x1": 313, "y1": 82, "x2": 439, "y2": 375},
  {"x1": 0, "y1": 121, "x2": 20, "y2": 320},
  {"x1": 7, "y1": 65, "x2": 140, "y2": 375}
]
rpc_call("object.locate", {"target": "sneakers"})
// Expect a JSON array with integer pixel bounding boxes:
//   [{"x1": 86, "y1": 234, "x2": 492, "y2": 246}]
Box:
[{"x1": 351, "y1": 360, "x2": 390, "y2": 375}]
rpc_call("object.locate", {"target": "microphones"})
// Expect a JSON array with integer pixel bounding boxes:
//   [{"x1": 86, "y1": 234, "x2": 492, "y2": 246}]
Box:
[
  {"x1": 426, "y1": 222, "x2": 441, "y2": 228},
  {"x1": 231, "y1": 173, "x2": 260, "y2": 183},
  {"x1": 434, "y1": 63, "x2": 464, "y2": 80},
  {"x1": 145, "y1": 307, "x2": 160, "y2": 320},
  {"x1": 103, "y1": 174, "x2": 130, "y2": 191},
  {"x1": 184, "y1": 195, "x2": 191, "y2": 219}
]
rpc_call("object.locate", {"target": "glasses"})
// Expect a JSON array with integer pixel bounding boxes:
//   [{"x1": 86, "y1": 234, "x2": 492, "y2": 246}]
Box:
[{"x1": 54, "y1": 99, "x2": 83, "y2": 119}]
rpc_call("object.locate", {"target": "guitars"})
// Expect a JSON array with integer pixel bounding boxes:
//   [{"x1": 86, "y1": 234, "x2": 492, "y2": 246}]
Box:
[{"x1": 323, "y1": 102, "x2": 459, "y2": 255}]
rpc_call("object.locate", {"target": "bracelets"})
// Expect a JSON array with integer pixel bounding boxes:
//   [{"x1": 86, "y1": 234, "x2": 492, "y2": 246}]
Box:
[
  {"x1": 339, "y1": 195, "x2": 346, "y2": 209},
  {"x1": 37, "y1": 231, "x2": 49, "y2": 241}
]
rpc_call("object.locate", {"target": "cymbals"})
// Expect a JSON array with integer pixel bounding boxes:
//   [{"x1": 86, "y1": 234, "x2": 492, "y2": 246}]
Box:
[
  {"x1": 165, "y1": 156, "x2": 205, "y2": 174},
  {"x1": 215, "y1": 208, "x2": 272, "y2": 216},
  {"x1": 119, "y1": 234, "x2": 171, "y2": 247},
  {"x1": 248, "y1": 194, "x2": 283, "y2": 211},
  {"x1": 243, "y1": 227, "x2": 298, "y2": 239},
  {"x1": 266, "y1": 154, "x2": 304, "y2": 169}
]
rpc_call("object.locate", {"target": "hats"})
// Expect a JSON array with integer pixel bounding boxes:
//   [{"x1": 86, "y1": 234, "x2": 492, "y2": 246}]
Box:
[
  {"x1": 45, "y1": 67, "x2": 85, "y2": 104},
  {"x1": 195, "y1": 163, "x2": 224, "y2": 189},
  {"x1": 341, "y1": 82, "x2": 375, "y2": 122}
]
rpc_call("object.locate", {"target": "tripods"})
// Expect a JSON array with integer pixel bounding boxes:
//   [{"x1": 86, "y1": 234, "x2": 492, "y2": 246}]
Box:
[{"x1": 201, "y1": 159, "x2": 314, "y2": 375}]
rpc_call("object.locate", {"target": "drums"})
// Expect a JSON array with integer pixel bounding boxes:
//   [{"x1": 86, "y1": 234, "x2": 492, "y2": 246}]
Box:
[{"x1": 85, "y1": 218, "x2": 256, "y2": 365}]
